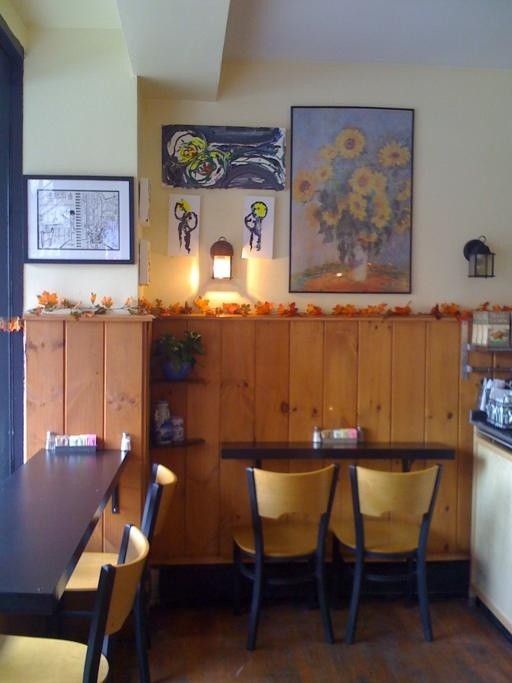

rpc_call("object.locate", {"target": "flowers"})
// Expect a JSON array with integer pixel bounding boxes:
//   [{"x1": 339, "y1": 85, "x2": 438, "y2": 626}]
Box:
[{"x1": 293, "y1": 128, "x2": 411, "y2": 282}]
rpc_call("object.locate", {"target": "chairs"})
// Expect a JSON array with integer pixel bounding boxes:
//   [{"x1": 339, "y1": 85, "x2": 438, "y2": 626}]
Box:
[
  {"x1": 232, "y1": 464, "x2": 339, "y2": 651},
  {"x1": 0, "y1": 524, "x2": 150, "y2": 683},
  {"x1": 328, "y1": 464, "x2": 442, "y2": 644},
  {"x1": 64, "y1": 462, "x2": 178, "y2": 683}
]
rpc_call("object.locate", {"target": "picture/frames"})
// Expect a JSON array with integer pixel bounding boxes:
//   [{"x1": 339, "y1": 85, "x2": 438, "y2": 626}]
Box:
[
  {"x1": 289, "y1": 106, "x2": 414, "y2": 293},
  {"x1": 22, "y1": 175, "x2": 135, "y2": 264}
]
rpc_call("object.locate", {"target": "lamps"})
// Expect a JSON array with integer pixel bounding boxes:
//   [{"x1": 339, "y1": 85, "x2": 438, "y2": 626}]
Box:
[
  {"x1": 211, "y1": 237, "x2": 233, "y2": 280},
  {"x1": 464, "y1": 235, "x2": 496, "y2": 277}
]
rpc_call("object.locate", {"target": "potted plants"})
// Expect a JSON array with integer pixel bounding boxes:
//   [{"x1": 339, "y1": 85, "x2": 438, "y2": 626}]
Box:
[{"x1": 151, "y1": 330, "x2": 206, "y2": 382}]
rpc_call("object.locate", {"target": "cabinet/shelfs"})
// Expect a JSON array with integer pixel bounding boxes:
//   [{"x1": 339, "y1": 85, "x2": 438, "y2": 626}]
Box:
[
  {"x1": 461, "y1": 321, "x2": 512, "y2": 380},
  {"x1": 149, "y1": 380, "x2": 204, "y2": 448},
  {"x1": 468, "y1": 426, "x2": 512, "y2": 643}
]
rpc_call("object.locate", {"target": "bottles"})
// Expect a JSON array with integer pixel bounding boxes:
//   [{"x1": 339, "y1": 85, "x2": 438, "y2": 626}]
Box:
[
  {"x1": 312, "y1": 441, "x2": 321, "y2": 449},
  {"x1": 312, "y1": 424, "x2": 322, "y2": 443},
  {"x1": 152, "y1": 400, "x2": 172, "y2": 445},
  {"x1": 44, "y1": 430, "x2": 55, "y2": 449},
  {"x1": 356, "y1": 425, "x2": 365, "y2": 442},
  {"x1": 170, "y1": 415, "x2": 184, "y2": 444},
  {"x1": 357, "y1": 441, "x2": 365, "y2": 450},
  {"x1": 120, "y1": 449, "x2": 128, "y2": 463},
  {"x1": 120, "y1": 431, "x2": 133, "y2": 451},
  {"x1": 45, "y1": 449, "x2": 55, "y2": 469}
]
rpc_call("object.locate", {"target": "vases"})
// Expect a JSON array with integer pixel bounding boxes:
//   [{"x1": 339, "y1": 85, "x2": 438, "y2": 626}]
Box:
[{"x1": 349, "y1": 242, "x2": 369, "y2": 282}]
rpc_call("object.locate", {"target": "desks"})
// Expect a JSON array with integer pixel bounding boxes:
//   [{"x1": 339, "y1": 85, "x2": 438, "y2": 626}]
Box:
[
  {"x1": 221, "y1": 442, "x2": 455, "y2": 472},
  {"x1": 0, "y1": 449, "x2": 129, "y2": 613}
]
